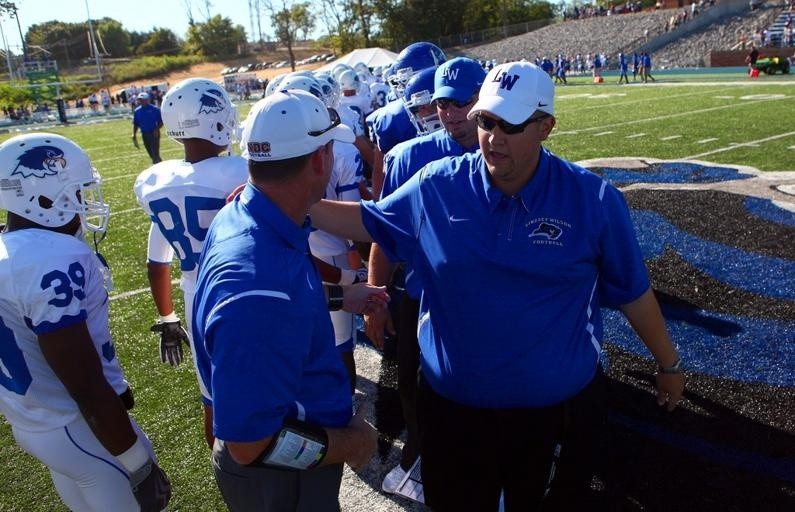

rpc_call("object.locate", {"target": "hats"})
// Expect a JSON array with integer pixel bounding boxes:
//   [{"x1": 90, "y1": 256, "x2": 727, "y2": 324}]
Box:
[
  {"x1": 136, "y1": 92, "x2": 149, "y2": 100},
  {"x1": 431, "y1": 57, "x2": 555, "y2": 125},
  {"x1": 241, "y1": 91, "x2": 356, "y2": 161}
]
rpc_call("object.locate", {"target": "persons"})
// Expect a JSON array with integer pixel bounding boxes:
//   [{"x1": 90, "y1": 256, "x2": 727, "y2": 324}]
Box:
[
  {"x1": 555, "y1": 1, "x2": 720, "y2": 44},
  {"x1": 0, "y1": 40, "x2": 688, "y2": 512},
  {"x1": 733, "y1": 1, "x2": 795, "y2": 78}
]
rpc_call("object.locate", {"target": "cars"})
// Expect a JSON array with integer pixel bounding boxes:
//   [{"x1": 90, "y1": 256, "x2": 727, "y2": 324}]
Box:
[{"x1": 220, "y1": 52, "x2": 337, "y2": 76}]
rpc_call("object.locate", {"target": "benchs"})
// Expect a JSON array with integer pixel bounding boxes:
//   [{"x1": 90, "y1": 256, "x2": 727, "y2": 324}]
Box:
[{"x1": 761, "y1": 7, "x2": 795, "y2": 48}]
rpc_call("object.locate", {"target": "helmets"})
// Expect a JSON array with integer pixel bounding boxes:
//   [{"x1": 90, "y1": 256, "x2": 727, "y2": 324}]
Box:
[
  {"x1": 0, "y1": 132, "x2": 110, "y2": 232},
  {"x1": 161, "y1": 43, "x2": 447, "y2": 145}
]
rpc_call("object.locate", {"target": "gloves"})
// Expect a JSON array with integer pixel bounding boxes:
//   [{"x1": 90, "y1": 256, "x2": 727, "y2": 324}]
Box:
[
  {"x1": 151, "y1": 317, "x2": 192, "y2": 365},
  {"x1": 129, "y1": 455, "x2": 172, "y2": 511}
]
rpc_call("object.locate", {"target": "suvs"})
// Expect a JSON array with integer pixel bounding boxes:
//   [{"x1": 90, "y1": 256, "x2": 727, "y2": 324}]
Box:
[{"x1": 748, "y1": 56, "x2": 790, "y2": 76}]
